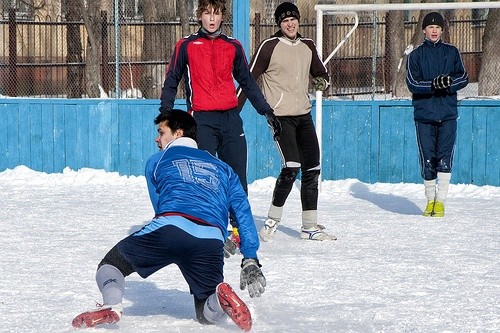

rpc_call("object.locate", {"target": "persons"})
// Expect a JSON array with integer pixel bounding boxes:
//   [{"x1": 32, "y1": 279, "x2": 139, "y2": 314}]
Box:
[
  {"x1": 236, "y1": 2, "x2": 337, "y2": 243},
  {"x1": 404, "y1": 11, "x2": 469, "y2": 218},
  {"x1": 159, "y1": 0, "x2": 283, "y2": 249},
  {"x1": 72, "y1": 108, "x2": 267, "y2": 333}
]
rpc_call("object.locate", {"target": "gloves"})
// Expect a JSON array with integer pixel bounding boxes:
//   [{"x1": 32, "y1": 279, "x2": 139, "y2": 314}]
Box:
[
  {"x1": 223, "y1": 238, "x2": 236, "y2": 258},
  {"x1": 311, "y1": 76, "x2": 326, "y2": 92},
  {"x1": 265, "y1": 112, "x2": 282, "y2": 141},
  {"x1": 240, "y1": 256, "x2": 266, "y2": 299},
  {"x1": 432, "y1": 73, "x2": 452, "y2": 90}
]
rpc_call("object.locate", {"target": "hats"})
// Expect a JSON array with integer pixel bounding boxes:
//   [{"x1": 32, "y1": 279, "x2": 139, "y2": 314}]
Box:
[
  {"x1": 273, "y1": 1, "x2": 300, "y2": 28},
  {"x1": 422, "y1": 12, "x2": 444, "y2": 31}
]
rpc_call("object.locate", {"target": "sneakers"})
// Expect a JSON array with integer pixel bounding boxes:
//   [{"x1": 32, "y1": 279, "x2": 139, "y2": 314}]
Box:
[
  {"x1": 215, "y1": 281, "x2": 252, "y2": 331},
  {"x1": 259, "y1": 217, "x2": 280, "y2": 242},
  {"x1": 71, "y1": 299, "x2": 124, "y2": 329},
  {"x1": 299, "y1": 225, "x2": 337, "y2": 241}
]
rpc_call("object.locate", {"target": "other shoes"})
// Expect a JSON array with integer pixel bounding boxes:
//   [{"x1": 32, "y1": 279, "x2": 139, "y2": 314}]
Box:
[
  {"x1": 422, "y1": 200, "x2": 444, "y2": 217},
  {"x1": 230, "y1": 226, "x2": 243, "y2": 255}
]
